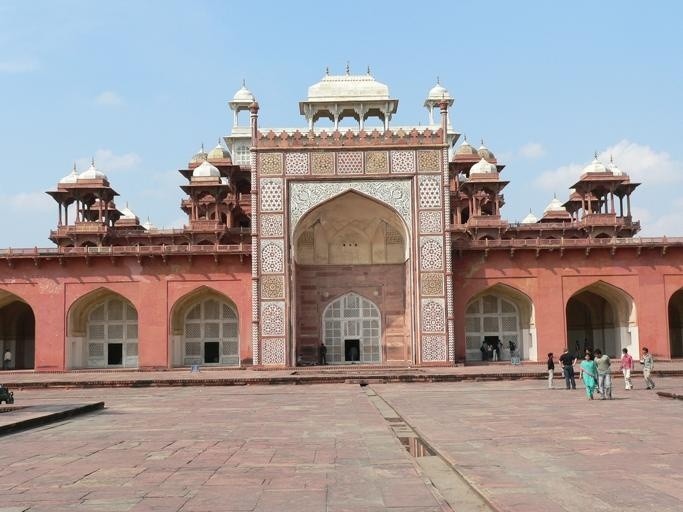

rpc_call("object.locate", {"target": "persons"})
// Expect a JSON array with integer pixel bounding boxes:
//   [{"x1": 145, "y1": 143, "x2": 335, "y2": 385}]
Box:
[
  {"x1": 480, "y1": 340, "x2": 503, "y2": 362},
  {"x1": 509, "y1": 341, "x2": 515, "y2": 357},
  {"x1": 548, "y1": 353, "x2": 555, "y2": 389},
  {"x1": 296, "y1": 353, "x2": 313, "y2": 366},
  {"x1": 3, "y1": 349, "x2": 12, "y2": 370},
  {"x1": 558, "y1": 337, "x2": 612, "y2": 401},
  {"x1": 642, "y1": 347, "x2": 655, "y2": 389},
  {"x1": 619, "y1": 348, "x2": 634, "y2": 391},
  {"x1": 320, "y1": 343, "x2": 328, "y2": 365}
]
